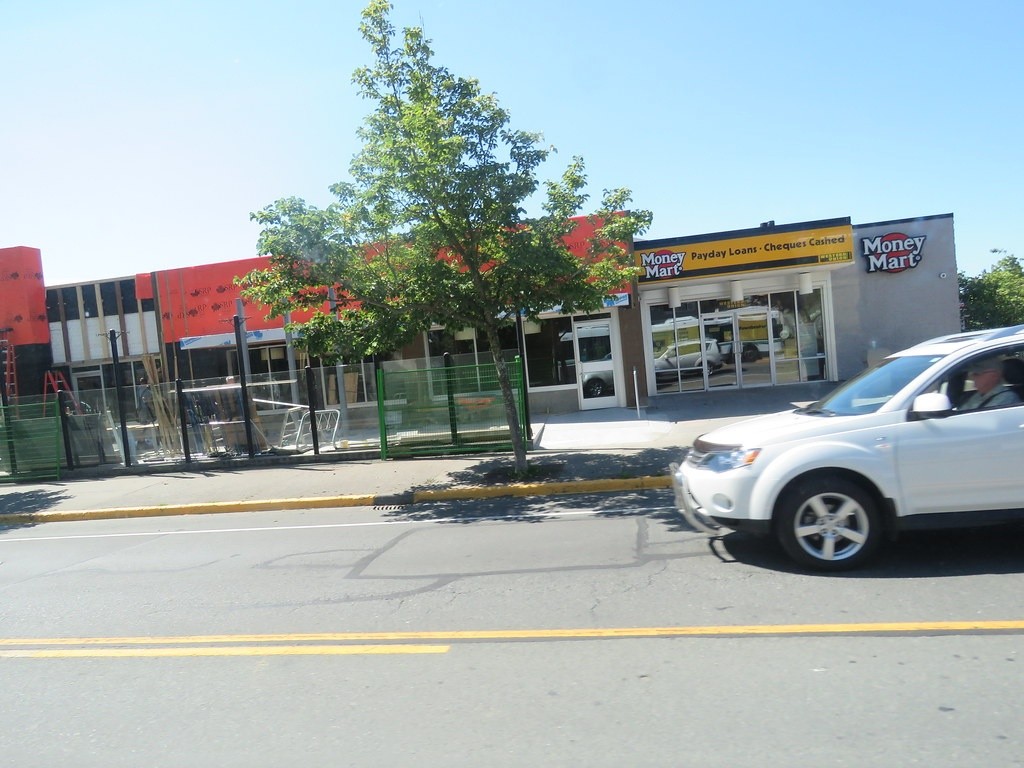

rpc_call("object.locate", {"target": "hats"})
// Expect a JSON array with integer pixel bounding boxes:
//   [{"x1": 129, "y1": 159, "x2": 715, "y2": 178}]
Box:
[{"x1": 967, "y1": 357, "x2": 1004, "y2": 374}]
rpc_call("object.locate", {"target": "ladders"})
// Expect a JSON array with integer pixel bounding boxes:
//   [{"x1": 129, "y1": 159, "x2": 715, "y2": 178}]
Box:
[
  {"x1": 0, "y1": 328, "x2": 19, "y2": 420},
  {"x1": 42, "y1": 369, "x2": 100, "y2": 458}
]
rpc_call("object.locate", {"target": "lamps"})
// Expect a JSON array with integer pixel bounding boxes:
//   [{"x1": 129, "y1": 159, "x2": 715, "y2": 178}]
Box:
[
  {"x1": 797, "y1": 271, "x2": 813, "y2": 296},
  {"x1": 667, "y1": 286, "x2": 681, "y2": 307},
  {"x1": 728, "y1": 280, "x2": 744, "y2": 302}
]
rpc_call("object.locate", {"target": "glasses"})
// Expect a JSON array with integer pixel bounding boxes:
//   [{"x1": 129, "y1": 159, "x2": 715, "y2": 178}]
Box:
[{"x1": 973, "y1": 371, "x2": 996, "y2": 375}]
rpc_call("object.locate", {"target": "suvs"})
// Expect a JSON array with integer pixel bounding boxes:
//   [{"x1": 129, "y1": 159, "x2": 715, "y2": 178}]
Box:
[
  {"x1": 582, "y1": 353, "x2": 613, "y2": 397},
  {"x1": 673, "y1": 323, "x2": 1023, "y2": 572},
  {"x1": 653, "y1": 338, "x2": 721, "y2": 381}
]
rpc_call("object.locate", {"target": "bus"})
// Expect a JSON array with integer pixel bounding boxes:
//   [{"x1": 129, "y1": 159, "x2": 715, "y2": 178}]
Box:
[
  {"x1": 652, "y1": 305, "x2": 785, "y2": 363},
  {"x1": 557, "y1": 324, "x2": 611, "y2": 381}
]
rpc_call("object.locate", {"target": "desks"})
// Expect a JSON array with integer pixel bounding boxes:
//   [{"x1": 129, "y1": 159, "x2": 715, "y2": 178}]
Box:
[{"x1": 259, "y1": 406, "x2": 307, "y2": 449}]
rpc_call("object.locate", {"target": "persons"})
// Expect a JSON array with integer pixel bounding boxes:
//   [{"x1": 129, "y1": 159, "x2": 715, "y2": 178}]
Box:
[
  {"x1": 957, "y1": 357, "x2": 1020, "y2": 409},
  {"x1": 226, "y1": 376, "x2": 235, "y2": 384},
  {"x1": 136, "y1": 378, "x2": 156, "y2": 446}
]
